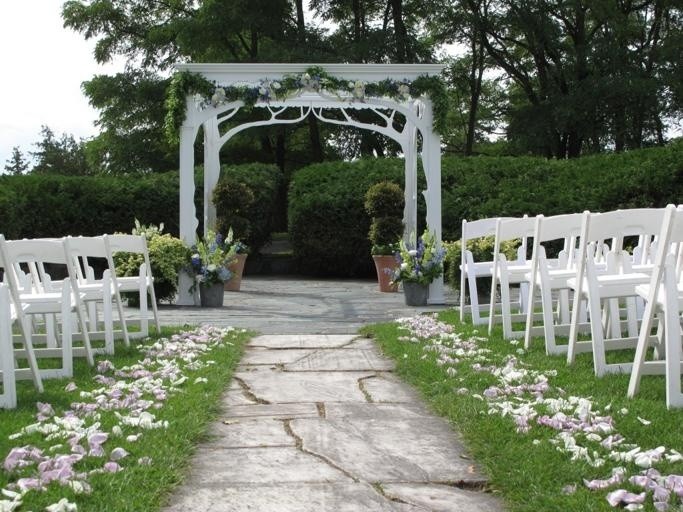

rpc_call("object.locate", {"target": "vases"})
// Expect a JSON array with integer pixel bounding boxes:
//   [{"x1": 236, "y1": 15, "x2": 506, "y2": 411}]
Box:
[
  {"x1": 200, "y1": 280, "x2": 223, "y2": 309},
  {"x1": 401, "y1": 279, "x2": 428, "y2": 306}
]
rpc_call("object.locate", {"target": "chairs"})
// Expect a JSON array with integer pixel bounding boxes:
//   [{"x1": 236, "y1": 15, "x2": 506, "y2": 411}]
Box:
[{"x1": 0, "y1": 234, "x2": 161, "y2": 412}]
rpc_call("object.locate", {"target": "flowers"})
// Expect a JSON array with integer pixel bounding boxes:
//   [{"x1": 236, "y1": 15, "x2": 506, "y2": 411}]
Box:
[
  {"x1": 181, "y1": 230, "x2": 239, "y2": 296},
  {"x1": 396, "y1": 231, "x2": 447, "y2": 288},
  {"x1": 104, "y1": 216, "x2": 188, "y2": 306}
]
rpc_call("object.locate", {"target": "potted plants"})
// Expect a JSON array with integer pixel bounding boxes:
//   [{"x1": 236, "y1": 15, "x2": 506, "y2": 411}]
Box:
[
  {"x1": 360, "y1": 180, "x2": 406, "y2": 293},
  {"x1": 211, "y1": 177, "x2": 252, "y2": 292}
]
